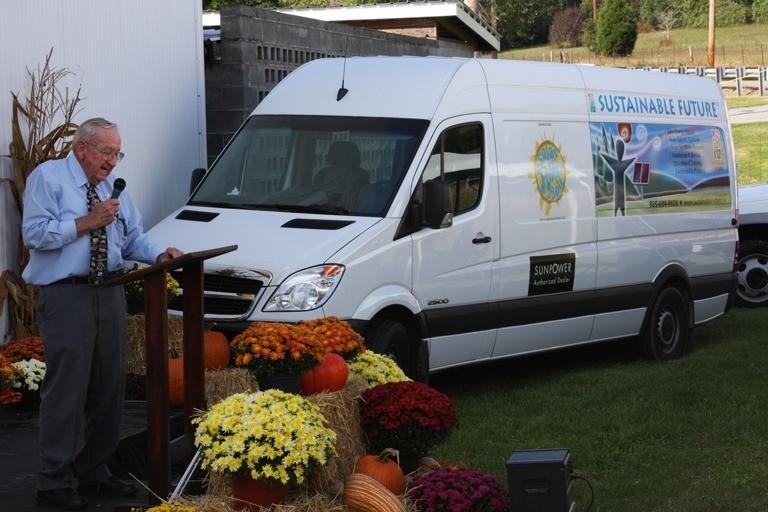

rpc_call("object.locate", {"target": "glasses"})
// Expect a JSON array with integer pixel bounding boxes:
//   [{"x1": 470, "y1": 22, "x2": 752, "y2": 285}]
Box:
[{"x1": 79, "y1": 139, "x2": 124, "y2": 161}]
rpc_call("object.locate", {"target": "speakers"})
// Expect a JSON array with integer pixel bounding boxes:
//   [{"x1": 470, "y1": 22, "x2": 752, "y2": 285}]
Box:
[{"x1": 506, "y1": 448, "x2": 578, "y2": 512}]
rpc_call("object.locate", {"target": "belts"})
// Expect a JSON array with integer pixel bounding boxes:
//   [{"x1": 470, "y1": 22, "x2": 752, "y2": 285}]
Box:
[{"x1": 56, "y1": 272, "x2": 117, "y2": 285}]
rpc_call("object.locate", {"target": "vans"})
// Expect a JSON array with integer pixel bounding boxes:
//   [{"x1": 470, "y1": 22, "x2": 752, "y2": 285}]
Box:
[{"x1": 144, "y1": 57, "x2": 740, "y2": 390}]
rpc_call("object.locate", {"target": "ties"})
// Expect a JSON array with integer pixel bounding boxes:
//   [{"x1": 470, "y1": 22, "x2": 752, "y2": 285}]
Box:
[{"x1": 84, "y1": 182, "x2": 108, "y2": 287}]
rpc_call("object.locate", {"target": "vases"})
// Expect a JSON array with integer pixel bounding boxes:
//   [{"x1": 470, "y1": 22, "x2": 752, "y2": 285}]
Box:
[
  {"x1": 380, "y1": 427, "x2": 422, "y2": 473},
  {"x1": 128, "y1": 297, "x2": 145, "y2": 315},
  {"x1": 232, "y1": 473, "x2": 292, "y2": 512},
  {"x1": 255, "y1": 374, "x2": 298, "y2": 395},
  {"x1": 23, "y1": 391, "x2": 40, "y2": 412}
]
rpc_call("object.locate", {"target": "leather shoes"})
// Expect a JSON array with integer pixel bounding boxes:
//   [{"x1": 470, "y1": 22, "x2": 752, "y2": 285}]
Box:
[
  {"x1": 37, "y1": 488, "x2": 87, "y2": 508},
  {"x1": 77, "y1": 480, "x2": 138, "y2": 497}
]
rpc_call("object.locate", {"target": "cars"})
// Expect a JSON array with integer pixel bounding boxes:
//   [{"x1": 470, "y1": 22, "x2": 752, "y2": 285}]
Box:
[{"x1": 733, "y1": 183, "x2": 768, "y2": 309}]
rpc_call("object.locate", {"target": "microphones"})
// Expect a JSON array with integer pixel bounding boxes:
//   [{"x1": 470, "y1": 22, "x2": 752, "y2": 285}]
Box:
[{"x1": 111, "y1": 177, "x2": 127, "y2": 204}]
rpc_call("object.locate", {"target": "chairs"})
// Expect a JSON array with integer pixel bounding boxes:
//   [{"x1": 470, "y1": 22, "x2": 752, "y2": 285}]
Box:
[{"x1": 307, "y1": 140, "x2": 370, "y2": 212}]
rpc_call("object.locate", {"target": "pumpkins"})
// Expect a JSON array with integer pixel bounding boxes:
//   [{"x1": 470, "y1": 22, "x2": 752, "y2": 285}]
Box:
[
  {"x1": 355, "y1": 448, "x2": 405, "y2": 495},
  {"x1": 326, "y1": 473, "x2": 408, "y2": 512},
  {"x1": 168, "y1": 347, "x2": 184, "y2": 408},
  {"x1": 205, "y1": 322, "x2": 230, "y2": 370},
  {"x1": 297, "y1": 353, "x2": 348, "y2": 394}
]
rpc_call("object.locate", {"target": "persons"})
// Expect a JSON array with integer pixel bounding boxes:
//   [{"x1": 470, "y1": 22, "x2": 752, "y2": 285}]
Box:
[{"x1": 20, "y1": 118, "x2": 183, "y2": 510}]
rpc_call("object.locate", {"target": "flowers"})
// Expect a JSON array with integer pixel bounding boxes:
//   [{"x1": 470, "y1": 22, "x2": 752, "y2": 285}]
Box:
[
  {"x1": 190, "y1": 387, "x2": 339, "y2": 485},
  {"x1": 405, "y1": 465, "x2": 508, "y2": 511},
  {"x1": 359, "y1": 381, "x2": 459, "y2": 445},
  {"x1": 0, "y1": 336, "x2": 47, "y2": 403},
  {"x1": 145, "y1": 503, "x2": 196, "y2": 512},
  {"x1": 346, "y1": 347, "x2": 414, "y2": 393},
  {"x1": 125, "y1": 265, "x2": 180, "y2": 303},
  {"x1": 227, "y1": 313, "x2": 365, "y2": 375}
]
